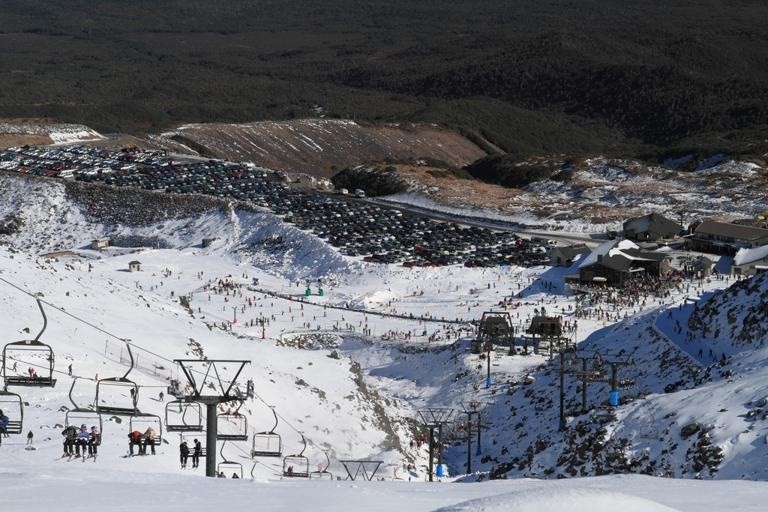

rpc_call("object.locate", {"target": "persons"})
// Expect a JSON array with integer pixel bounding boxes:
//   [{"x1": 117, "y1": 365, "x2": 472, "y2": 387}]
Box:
[
  {"x1": 217, "y1": 471, "x2": 226, "y2": 478},
  {"x1": 179, "y1": 442, "x2": 190, "y2": 468},
  {"x1": 288, "y1": 465, "x2": 293, "y2": 473},
  {"x1": 127, "y1": 430, "x2": 143, "y2": 457},
  {"x1": 25, "y1": 431, "x2": 33, "y2": 444},
  {"x1": 86, "y1": 425, "x2": 99, "y2": 460},
  {"x1": 26, "y1": 366, "x2": 39, "y2": 377},
  {"x1": 0, "y1": 409, "x2": 9, "y2": 438},
  {"x1": 123, "y1": 263, "x2": 746, "y2": 367},
  {"x1": 157, "y1": 391, "x2": 164, "y2": 401},
  {"x1": 231, "y1": 473, "x2": 239, "y2": 479},
  {"x1": 74, "y1": 423, "x2": 88, "y2": 458},
  {"x1": 61, "y1": 423, "x2": 77, "y2": 458},
  {"x1": 191, "y1": 439, "x2": 202, "y2": 469},
  {"x1": 11, "y1": 361, "x2": 18, "y2": 372},
  {"x1": 68, "y1": 363, "x2": 73, "y2": 377},
  {"x1": 129, "y1": 387, "x2": 135, "y2": 400},
  {"x1": 141, "y1": 426, "x2": 156, "y2": 455}
]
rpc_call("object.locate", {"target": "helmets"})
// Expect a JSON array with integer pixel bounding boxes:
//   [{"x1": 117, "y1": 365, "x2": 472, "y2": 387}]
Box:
[
  {"x1": 82, "y1": 424, "x2": 86, "y2": 429},
  {"x1": 92, "y1": 426, "x2": 97, "y2": 429}
]
